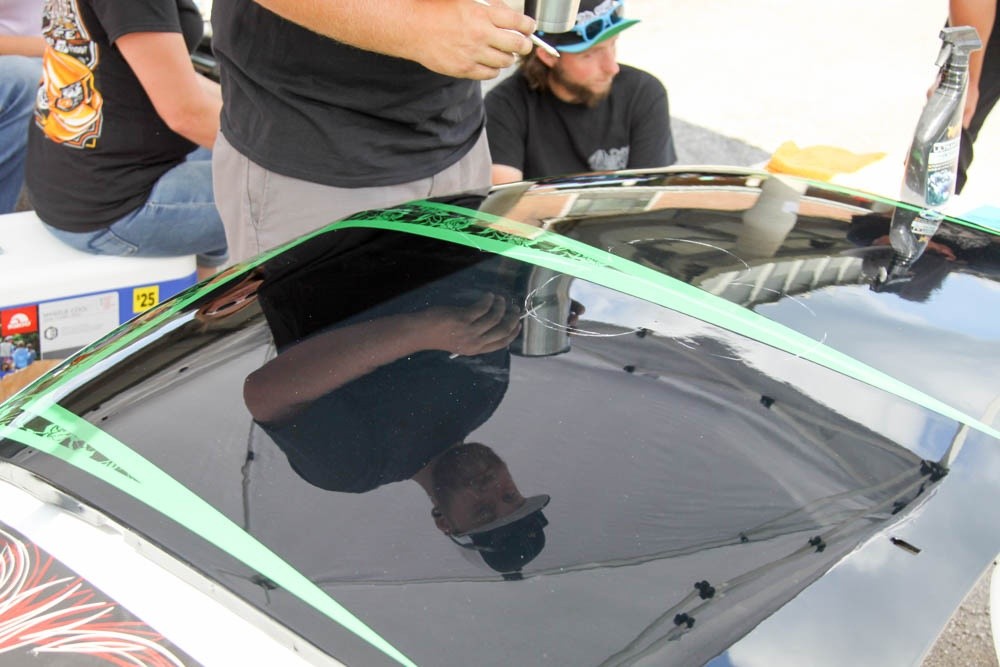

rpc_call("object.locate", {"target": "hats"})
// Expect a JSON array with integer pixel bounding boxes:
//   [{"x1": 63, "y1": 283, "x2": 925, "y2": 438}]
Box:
[
  {"x1": 525, "y1": 1, "x2": 641, "y2": 54},
  {"x1": 459, "y1": 495, "x2": 554, "y2": 576}
]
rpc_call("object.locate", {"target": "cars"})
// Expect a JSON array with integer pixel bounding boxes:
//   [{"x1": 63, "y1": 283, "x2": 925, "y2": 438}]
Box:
[{"x1": 0, "y1": 163, "x2": 1000, "y2": 667}]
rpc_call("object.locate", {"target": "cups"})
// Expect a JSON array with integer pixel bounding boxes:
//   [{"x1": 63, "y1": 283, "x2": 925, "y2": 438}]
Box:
[
  {"x1": 523, "y1": 0, "x2": 581, "y2": 34},
  {"x1": 507, "y1": 263, "x2": 573, "y2": 359}
]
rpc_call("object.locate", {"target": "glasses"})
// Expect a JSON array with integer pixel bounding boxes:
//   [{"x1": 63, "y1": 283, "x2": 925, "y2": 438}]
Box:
[
  {"x1": 449, "y1": 512, "x2": 549, "y2": 554},
  {"x1": 541, "y1": 2, "x2": 625, "y2": 44}
]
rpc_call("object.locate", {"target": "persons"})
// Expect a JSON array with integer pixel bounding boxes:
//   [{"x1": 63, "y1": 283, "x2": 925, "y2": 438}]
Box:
[
  {"x1": 483, "y1": 0, "x2": 678, "y2": 184},
  {"x1": 243, "y1": 271, "x2": 584, "y2": 575},
  {"x1": 903, "y1": 0, "x2": 1000, "y2": 195},
  {"x1": 845, "y1": 207, "x2": 1000, "y2": 302},
  {"x1": 0, "y1": 0, "x2": 230, "y2": 284},
  {"x1": 210, "y1": 0, "x2": 537, "y2": 266}
]
